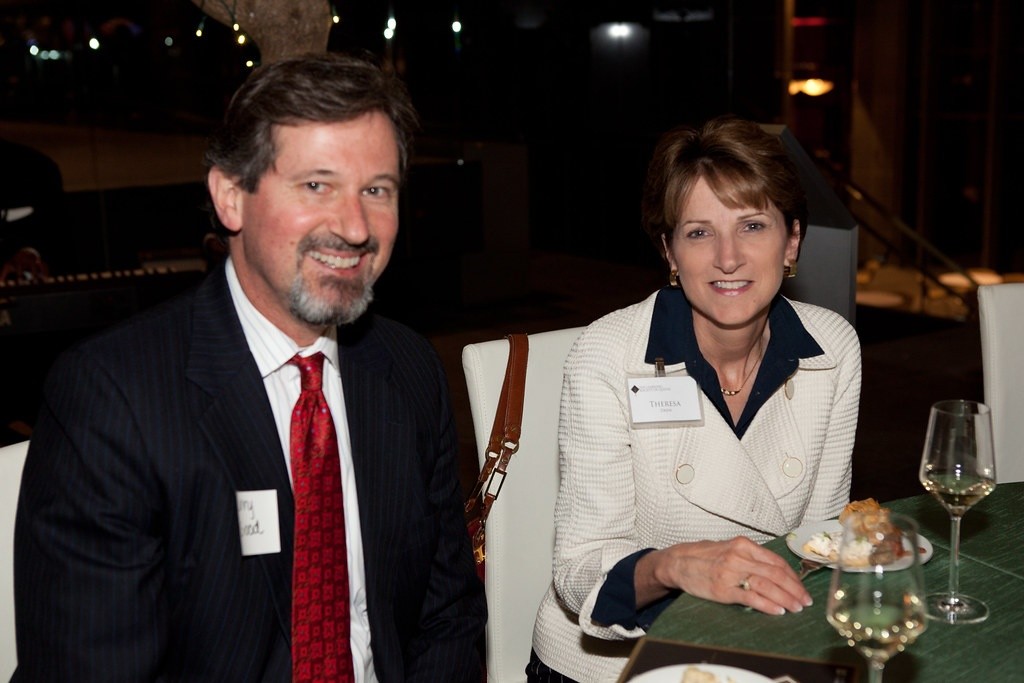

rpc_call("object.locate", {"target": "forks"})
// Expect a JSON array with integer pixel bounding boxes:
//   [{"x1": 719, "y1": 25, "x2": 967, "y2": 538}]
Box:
[{"x1": 740, "y1": 556, "x2": 836, "y2": 613}]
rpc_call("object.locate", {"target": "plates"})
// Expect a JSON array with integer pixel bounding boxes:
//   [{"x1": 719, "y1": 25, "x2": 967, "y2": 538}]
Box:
[
  {"x1": 627, "y1": 663, "x2": 780, "y2": 683},
  {"x1": 786, "y1": 520, "x2": 933, "y2": 573}
]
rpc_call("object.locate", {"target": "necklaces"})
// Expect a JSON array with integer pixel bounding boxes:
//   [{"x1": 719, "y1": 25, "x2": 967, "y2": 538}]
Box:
[{"x1": 720, "y1": 342, "x2": 762, "y2": 396}]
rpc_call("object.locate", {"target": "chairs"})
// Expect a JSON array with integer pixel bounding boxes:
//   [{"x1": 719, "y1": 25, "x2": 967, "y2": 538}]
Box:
[
  {"x1": 460, "y1": 326, "x2": 589, "y2": 683},
  {"x1": 977, "y1": 282, "x2": 1024, "y2": 484}
]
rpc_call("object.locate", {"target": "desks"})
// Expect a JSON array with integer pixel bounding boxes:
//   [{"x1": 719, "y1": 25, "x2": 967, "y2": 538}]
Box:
[{"x1": 615, "y1": 480, "x2": 1024, "y2": 683}]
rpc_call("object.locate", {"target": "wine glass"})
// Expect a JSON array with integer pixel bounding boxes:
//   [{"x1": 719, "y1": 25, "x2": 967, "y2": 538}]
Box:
[
  {"x1": 827, "y1": 511, "x2": 930, "y2": 683},
  {"x1": 919, "y1": 399, "x2": 998, "y2": 626}
]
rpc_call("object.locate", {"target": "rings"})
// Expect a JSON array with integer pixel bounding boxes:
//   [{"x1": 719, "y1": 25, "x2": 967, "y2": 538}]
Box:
[{"x1": 741, "y1": 574, "x2": 755, "y2": 590}]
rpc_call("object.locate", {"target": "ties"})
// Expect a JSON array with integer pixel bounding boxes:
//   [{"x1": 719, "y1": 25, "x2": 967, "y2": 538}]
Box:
[{"x1": 284, "y1": 352, "x2": 355, "y2": 683}]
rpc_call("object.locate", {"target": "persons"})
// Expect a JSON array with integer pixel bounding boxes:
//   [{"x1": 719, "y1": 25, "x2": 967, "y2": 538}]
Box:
[
  {"x1": 525, "y1": 119, "x2": 864, "y2": 683},
  {"x1": 14, "y1": 51, "x2": 478, "y2": 683},
  {"x1": 0, "y1": 135, "x2": 65, "y2": 278}
]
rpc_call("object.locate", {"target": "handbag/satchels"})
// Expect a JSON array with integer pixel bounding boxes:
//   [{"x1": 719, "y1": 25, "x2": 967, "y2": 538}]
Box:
[{"x1": 464, "y1": 502, "x2": 488, "y2": 581}]
